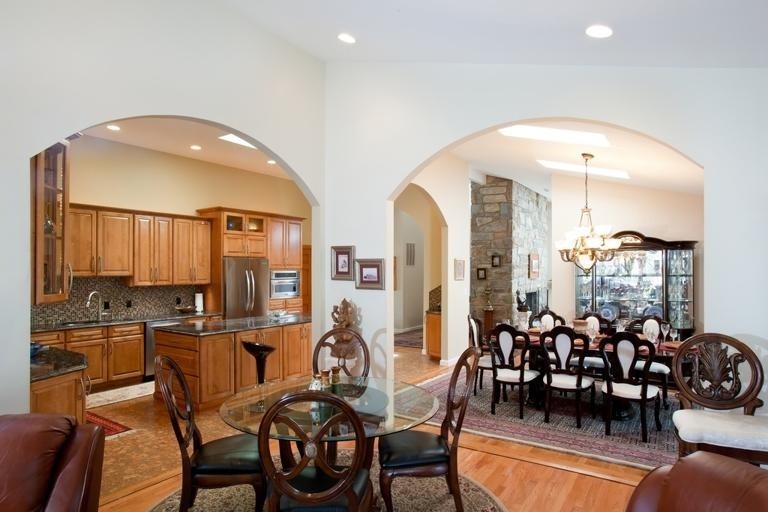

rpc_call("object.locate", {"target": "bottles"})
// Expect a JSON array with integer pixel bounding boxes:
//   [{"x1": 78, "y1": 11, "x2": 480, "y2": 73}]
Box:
[
  {"x1": 581, "y1": 255, "x2": 660, "y2": 309},
  {"x1": 307, "y1": 366, "x2": 346, "y2": 407},
  {"x1": 309, "y1": 408, "x2": 346, "y2": 429},
  {"x1": 44, "y1": 214, "x2": 53, "y2": 233}
]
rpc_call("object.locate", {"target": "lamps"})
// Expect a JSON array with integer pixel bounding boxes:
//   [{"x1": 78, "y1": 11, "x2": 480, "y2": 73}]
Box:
[{"x1": 557, "y1": 152, "x2": 622, "y2": 279}]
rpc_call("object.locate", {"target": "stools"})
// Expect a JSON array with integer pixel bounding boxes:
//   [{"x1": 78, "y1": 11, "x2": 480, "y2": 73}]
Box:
[{"x1": 241, "y1": 340, "x2": 275, "y2": 384}]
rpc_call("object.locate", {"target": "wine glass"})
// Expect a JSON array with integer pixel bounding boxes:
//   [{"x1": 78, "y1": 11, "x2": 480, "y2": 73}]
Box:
[
  {"x1": 587, "y1": 327, "x2": 597, "y2": 345},
  {"x1": 645, "y1": 322, "x2": 678, "y2": 350},
  {"x1": 668, "y1": 250, "x2": 690, "y2": 329}
]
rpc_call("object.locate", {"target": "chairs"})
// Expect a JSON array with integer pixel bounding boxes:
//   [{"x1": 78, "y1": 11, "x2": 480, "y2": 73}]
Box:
[
  {"x1": 466, "y1": 306, "x2": 671, "y2": 442},
  {"x1": 377, "y1": 347, "x2": 482, "y2": 512},
  {"x1": 669, "y1": 329, "x2": 765, "y2": 416},
  {"x1": 625, "y1": 450, "x2": 767, "y2": 512},
  {"x1": 295, "y1": 326, "x2": 372, "y2": 458},
  {"x1": 257, "y1": 392, "x2": 373, "y2": 512},
  {"x1": 151, "y1": 355, "x2": 269, "y2": 511},
  {"x1": 0, "y1": 413, "x2": 106, "y2": 511}
]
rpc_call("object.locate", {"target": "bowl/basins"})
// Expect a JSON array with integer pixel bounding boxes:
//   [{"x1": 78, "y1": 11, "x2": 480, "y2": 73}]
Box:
[{"x1": 175, "y1": 305, "x2": 198, "y2": 312}]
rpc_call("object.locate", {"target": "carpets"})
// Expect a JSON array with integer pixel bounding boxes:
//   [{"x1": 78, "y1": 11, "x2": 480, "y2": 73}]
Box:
[
  {"x1": 394, "y1": 328, "x2": 424, "y2": 348},
  {"x1": 84, "y1": 408, "x2": 133, "y2": 440},
  {"x1": 150, "y1": 448, "x2": 509, "y2": 512},
  {"x1": 392, "y1": 364, "x2": 681, "y2": 469}
]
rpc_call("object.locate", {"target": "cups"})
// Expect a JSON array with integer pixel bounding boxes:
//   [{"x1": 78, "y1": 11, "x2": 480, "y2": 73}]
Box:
[{"x1": 616, "y1": 318, "x2": 625, "y2": 332}]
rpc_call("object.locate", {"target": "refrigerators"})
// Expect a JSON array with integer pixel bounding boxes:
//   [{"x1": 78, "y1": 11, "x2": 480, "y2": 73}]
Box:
[{"x1": 224, "y1": 254, "x2": 270, "y2": 319}]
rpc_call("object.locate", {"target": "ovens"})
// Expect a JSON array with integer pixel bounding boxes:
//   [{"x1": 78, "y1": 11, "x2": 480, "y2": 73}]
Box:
[
  {"x1": 270, "y1": 269, "x2": 300, "y2": 299},
  {"x1": 145, "y1": 321, "x2": 181, "y2": 378}
]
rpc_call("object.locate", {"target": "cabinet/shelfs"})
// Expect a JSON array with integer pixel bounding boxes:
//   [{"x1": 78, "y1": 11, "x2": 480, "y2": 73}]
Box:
[
  {"x1": 116, "y1": 215, "x2": 172, "y2": 286},
  {"x1": 268, "y1": 214, "x2": 303, "y2": 269},
  {"x1": 269, "y1": 298, "x2": 304, "y2": 316},
  {"x1": 172, "y1": 217, "x2": 212, "y2": 286},
  {"x1": 30, "y1": 365, "x2": 88, "y2": 427},
  {"x1": 197, "y1": 211, "x2": 268, "y2": 257},
  {"x1": 575, "y1": 231, "x2": 697, "y2": 336},
  {"x1": 30, "y1": 331, "x2": 66, "y2": 351},
  {"x1": 425, "y1": 311, "x2": 442, "y2": 360},
  {"x1": 64, "y1": 205, "x2": 135, "y2": 277},
  {"x1": 31, "y1": 140, "x2": 70, "y2": 305},
  {"x1": 66, "y1": 322, "x2": 147, "y2": 386}
]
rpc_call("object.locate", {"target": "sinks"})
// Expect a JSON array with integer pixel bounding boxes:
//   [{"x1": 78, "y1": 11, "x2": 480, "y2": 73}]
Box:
[{"x1": 58, "y1": 320, "x2": 108, "y2": 327}]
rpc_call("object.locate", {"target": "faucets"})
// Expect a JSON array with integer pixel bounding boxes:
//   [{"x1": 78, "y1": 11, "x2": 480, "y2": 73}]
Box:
[{"x1": 85, "y1": 290, "x2": 101, "y2": 321}]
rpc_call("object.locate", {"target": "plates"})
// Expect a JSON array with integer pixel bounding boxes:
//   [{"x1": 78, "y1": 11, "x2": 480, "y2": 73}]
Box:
[
  {"x1": 516, "y1": 336, "x2": 539, "y2": 342},
  {"x1": 664, "y1": 341, "x2": 696, "y2": 350},
  {"x1": 600, "y1": 305, "x2": 616, "y2": 321},
  {"x1": 574, "y1": 340, "x2": 584, "y2": 346},
  {"x1": 644, "y1": 306, "x2": 663, "y2": 319}
]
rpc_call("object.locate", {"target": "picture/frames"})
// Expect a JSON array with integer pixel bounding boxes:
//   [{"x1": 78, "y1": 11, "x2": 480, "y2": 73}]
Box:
[
  {"x1": 356, "y1": 257, "x2": 386, "y2": 291},
  {"x1": 476, "y1": 268, "x2": 487, "y2": 281},
  {"x1": 490, "y1": 254, "x2": 502, "y2": 268},
  {"x1": 330, "y1": 245, "x2": 355, "y2": 281}
]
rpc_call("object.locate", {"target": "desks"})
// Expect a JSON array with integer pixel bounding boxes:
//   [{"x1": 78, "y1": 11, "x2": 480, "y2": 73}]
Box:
[
  {"x1": 152, "y1": 313, "x2": 312, "y2": 414},
  {"x1": 673, "y1": 409, "x2": 768, "y2": 465}
]
